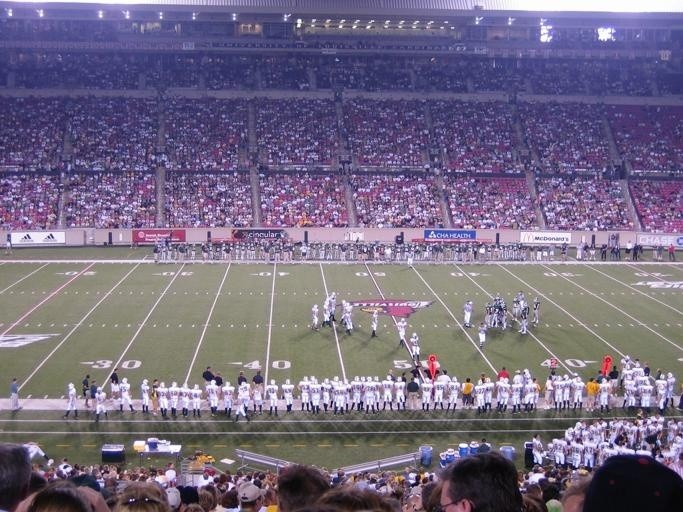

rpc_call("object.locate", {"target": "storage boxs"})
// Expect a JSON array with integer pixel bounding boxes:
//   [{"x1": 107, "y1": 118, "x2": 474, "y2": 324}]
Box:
[
  {"x1": 132, "y1": 438, "x2": 158, "y2": 451},
  {"x1": 101, "y1": 444, "x2": 124, "y2": 457}
]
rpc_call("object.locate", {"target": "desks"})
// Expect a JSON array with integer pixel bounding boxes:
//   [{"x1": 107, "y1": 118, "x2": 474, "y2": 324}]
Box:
[{"x1": 136, "y1": 443, "x2": 182, "y2": 466}]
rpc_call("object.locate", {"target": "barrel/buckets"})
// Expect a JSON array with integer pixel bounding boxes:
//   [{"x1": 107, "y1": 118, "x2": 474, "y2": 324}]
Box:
[
  {"x1": 500, "y1": 446, "x2": 515, "y2": 462},
  {"x1": 470, "y1": 441, "x2": 478, "y2": 455},
  {"x1": 458, "y1": 443, "x2": 469, "y2": 456}
]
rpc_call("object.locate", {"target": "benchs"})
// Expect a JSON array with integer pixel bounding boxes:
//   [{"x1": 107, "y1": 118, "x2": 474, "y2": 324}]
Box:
[
  {"x1": 330, "y1": 451, "x2": 420, "y2": 477},
  {"x1": 234, "y1": 448, "x2": 297, "y2": 475}
]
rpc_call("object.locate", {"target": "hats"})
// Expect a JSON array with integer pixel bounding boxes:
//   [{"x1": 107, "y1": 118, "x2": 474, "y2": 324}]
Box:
[{"x1": 237, "y1": 481, "x2": 267, "y2": 503}]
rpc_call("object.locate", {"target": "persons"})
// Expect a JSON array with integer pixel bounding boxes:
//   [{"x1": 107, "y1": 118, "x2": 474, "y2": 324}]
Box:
[
  {"x1": 311, "y1": 292, "x2": 357, "y2": 335},
  {"x1": 462, "y1": 290, "x2": 540, "y2": 347},
  {"x1": 369, "y1": 309, "x2": 378, "y2": 336},
  {"x1": 409, "y1": 332, "x2": 421, "y2": 363},
  {"x1": 395, "y1": 317, "x2": 408, "y2": 347}
]
